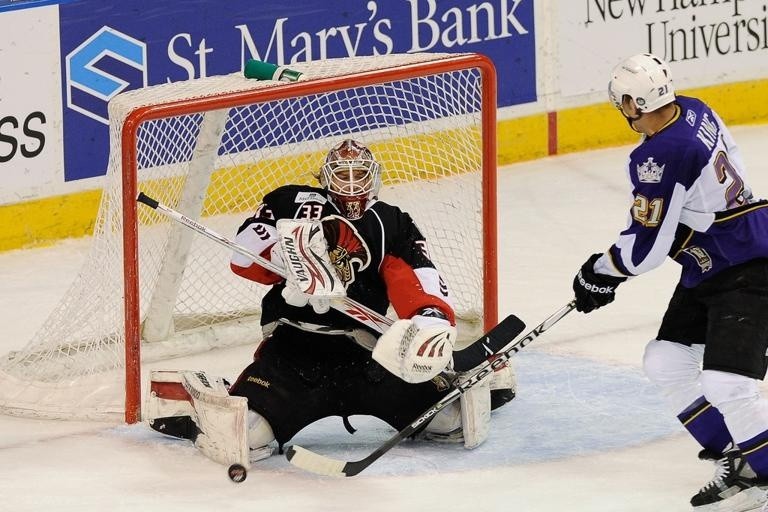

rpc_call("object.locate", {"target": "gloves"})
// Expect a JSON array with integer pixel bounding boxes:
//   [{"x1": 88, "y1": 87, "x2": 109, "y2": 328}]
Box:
[{"x1": 572, "y1": 252, "x2": 627, "y2": 313}]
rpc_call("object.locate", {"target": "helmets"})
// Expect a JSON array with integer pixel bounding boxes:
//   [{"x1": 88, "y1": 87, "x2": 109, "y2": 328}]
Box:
[
  {"x1": 607, "y1": 53, "x2": 676, "y2": 113},
  {"x1": 317, "y1": 139, "x2": 381, "y2": 221}
]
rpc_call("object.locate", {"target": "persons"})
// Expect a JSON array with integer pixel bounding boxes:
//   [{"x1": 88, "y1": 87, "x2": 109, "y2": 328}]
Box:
[
  {"x1": 574, "y1": 54, "x2": 768, "y2": 506},
  {"x1": 145, "y1": 141, "x2": 514, "y2": 466}
]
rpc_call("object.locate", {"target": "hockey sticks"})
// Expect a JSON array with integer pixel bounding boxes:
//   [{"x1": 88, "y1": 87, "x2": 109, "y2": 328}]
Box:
[
  {"x1": 285, "y1": 298, "x2": 578, "y2": 479},
  {"x1": 137, "y1": 192, "x2": 526, "y2": 374}
]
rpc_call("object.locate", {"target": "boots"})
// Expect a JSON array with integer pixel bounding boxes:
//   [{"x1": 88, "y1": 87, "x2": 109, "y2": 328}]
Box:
[{"x1": 689, "y1": 439, "x2": 758, "y2": 507}]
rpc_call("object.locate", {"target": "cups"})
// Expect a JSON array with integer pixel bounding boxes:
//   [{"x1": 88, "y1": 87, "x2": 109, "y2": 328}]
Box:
[{"x1": 244, "y1": 59, "x2": 305, "y2": 82}]
